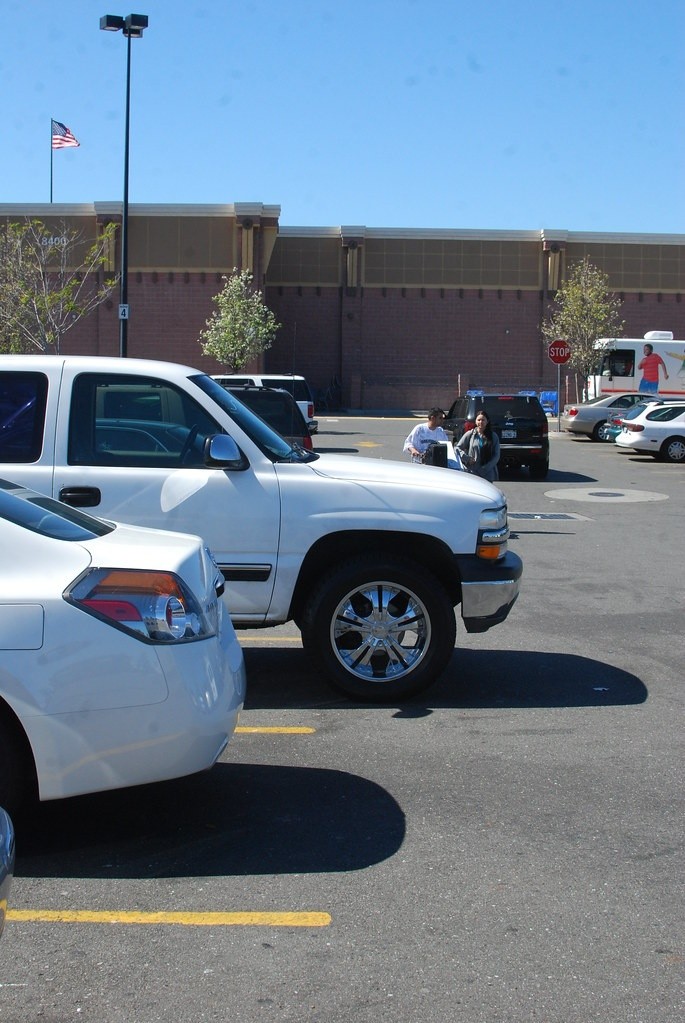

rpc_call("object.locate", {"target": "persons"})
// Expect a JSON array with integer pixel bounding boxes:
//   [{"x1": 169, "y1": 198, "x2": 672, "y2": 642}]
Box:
[
  {"x1": 402, "y1": 407, "x2": 449, "y2": 464},
  {"x1": 455, "y1": 411, "x2": 500, "y2": 484},
  {"x1": 637, "y1": 344, "x2": 669, "y2": 393},
  {"x1": 664, "y1": 349, "x2": 685, "y2": 369}
]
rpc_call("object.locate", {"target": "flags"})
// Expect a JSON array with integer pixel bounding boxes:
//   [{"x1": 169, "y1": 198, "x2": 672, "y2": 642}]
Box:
[{"x1": 51, "y1": 120, "x2": 80, "y2": 149}]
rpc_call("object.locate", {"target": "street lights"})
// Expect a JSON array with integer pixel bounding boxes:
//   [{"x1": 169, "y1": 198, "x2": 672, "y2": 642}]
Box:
[{"x1": 97, "y1": 13, "x2": 152, "y2": 359}]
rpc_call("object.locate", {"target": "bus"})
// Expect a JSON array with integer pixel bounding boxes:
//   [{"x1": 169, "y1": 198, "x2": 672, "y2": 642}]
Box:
[{"x1": 583, "y1": 330, "x2": 685, "y2": 407}]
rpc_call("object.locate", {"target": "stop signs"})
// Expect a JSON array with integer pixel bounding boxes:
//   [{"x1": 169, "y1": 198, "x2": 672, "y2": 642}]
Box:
[{"x1": 547, "y1": 340, "x2": 573, "y2": 364}]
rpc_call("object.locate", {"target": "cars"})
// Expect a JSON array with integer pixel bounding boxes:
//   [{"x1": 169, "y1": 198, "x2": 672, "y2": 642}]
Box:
[
  {"x1": 607, "y1": 396, "x2": 685, "y2": 464},
  {"x1": 561, "y1": 391, "x2": 663, "y2": 443},
  {"x1": 0, "y1": 473, "x2": 252, "y2": 834}
]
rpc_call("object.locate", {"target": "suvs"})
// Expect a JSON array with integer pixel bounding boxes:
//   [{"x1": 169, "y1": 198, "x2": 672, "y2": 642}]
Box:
[
  {"x1": 0, "y1": 349, "x2": 527, "y2": 705},
  {"x1": 83, "y1": 369, "x2": 319, "y2": 454},
  {"x1": 441, "y1": 388, "x2": 551, "y2": 482}
]
